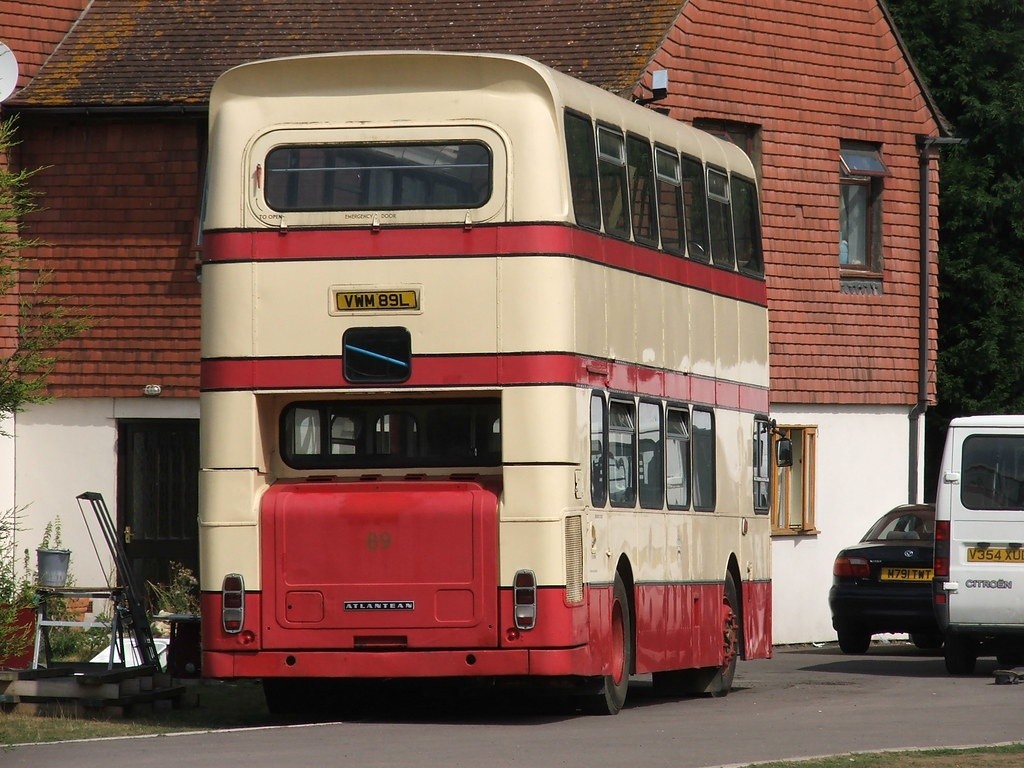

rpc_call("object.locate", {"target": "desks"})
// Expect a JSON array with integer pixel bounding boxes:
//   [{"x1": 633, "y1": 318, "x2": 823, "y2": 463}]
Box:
[{"x1": 32, "y1": 586, "x2": 129, "y2": 670}]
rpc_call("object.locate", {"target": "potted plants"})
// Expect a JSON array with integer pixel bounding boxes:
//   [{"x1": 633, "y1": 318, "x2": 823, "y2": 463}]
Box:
[{"x1": 35, "y1": 513, "x2": 71, "y2": 588}]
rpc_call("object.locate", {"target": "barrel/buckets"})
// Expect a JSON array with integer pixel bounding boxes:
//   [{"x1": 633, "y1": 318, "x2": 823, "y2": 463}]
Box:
[{"x1": 37, "y1": 549, "x2": 72, "y2": 587}]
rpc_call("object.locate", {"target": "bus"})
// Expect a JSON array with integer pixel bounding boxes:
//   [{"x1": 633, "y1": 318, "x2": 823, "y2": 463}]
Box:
[{"x1": 195, "y1": 46, "x2": 794, "y2": 718}]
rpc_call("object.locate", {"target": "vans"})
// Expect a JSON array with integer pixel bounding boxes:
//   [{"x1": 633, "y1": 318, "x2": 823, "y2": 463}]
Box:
[{"x1": 934, "y1": 415, "x2": 1024, "y2": 677}]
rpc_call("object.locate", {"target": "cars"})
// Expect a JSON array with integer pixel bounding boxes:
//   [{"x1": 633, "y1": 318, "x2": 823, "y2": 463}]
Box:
[{"x1": 829, "y1": 502, "x2": 943, "y2": 655}]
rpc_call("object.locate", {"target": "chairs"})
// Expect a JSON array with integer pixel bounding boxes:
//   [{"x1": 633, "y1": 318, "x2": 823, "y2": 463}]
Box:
[{"x1": 887, "y1": 531, "x2": 920, "y2": 539}]
